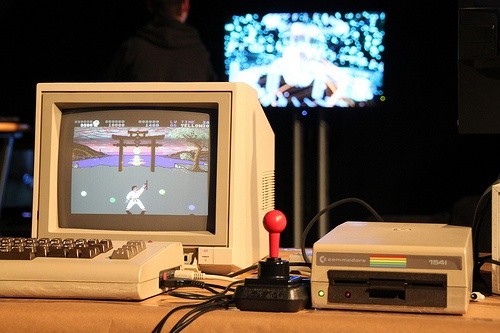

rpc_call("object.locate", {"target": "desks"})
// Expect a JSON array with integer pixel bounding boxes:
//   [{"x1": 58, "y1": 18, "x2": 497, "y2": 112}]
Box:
[{"x1": 0, "y1": 246, "x2": 500, "y2": 333}]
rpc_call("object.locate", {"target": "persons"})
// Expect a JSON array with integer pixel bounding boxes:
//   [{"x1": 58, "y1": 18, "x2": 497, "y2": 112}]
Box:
[{"x1": 119, "y1": 0, "x2": 217, "y2": 82}]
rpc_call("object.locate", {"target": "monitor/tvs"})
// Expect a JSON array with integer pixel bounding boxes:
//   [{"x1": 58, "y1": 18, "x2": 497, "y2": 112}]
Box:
[
  {"x1": 31, "y1": 82, "x2": 275, "y2": 275},
  {"x1": 221, "y1": 13, "x2": 385, "y2": 116}
]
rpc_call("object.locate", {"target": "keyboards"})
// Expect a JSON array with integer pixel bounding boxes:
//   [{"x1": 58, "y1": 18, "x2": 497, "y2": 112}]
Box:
[{"x1": 0, "y1": 236, "x2": 184, "y2": 301}]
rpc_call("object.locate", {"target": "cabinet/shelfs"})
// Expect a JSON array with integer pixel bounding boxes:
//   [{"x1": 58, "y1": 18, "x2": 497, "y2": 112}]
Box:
[{"x1": 458, "y1": 1, "x2": 500, "y2": 134}]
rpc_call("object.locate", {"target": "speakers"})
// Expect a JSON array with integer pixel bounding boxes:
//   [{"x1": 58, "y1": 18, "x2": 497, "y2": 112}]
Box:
[{"x1": 457, "y1": 0, "x2": 500, "y2": 134}]
